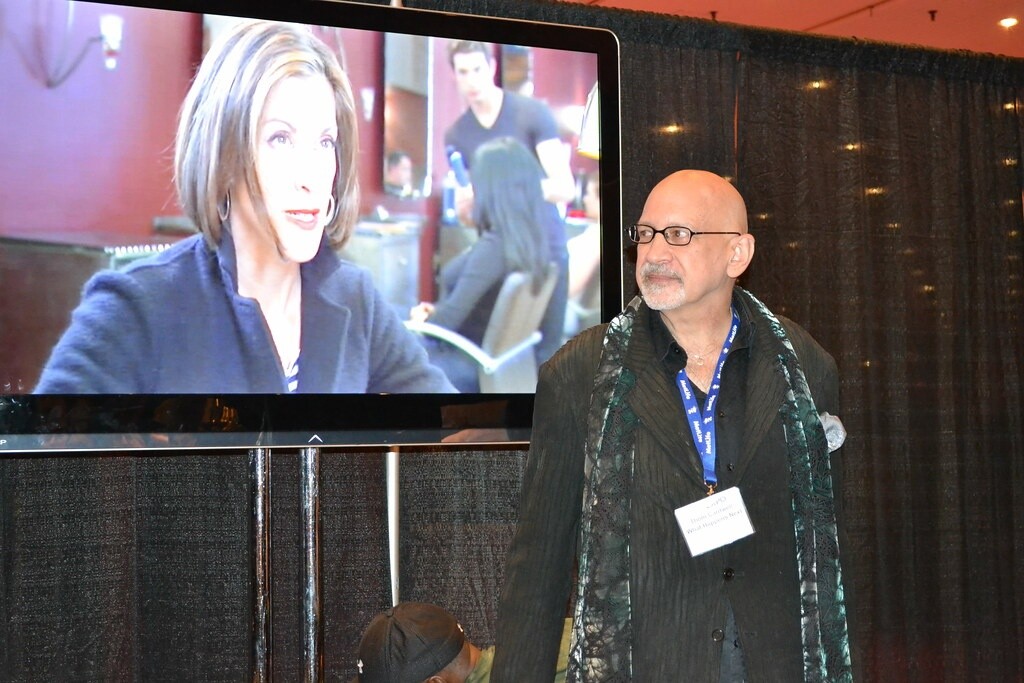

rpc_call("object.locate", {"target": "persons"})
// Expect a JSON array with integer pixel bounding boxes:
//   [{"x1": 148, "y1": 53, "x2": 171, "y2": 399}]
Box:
[
  {"x1": 565, "y1": 82, "x2": 599, "y2": 340},
  {"x1": 445, "y1": 41, "x2": 575, "y2": 363},
  {"x1": 410, "y1": 137, "x2": 548, "y2": 393},
  {"x1": 386, "y1": 152, "x2": 419, "y2": 198},
  {"x1": 34, "y1": 19, "x2": 459, "y2": 393},
  {"x1": 358, "y1": 602, "x2": 573, "y2": 683},
  {"x1": 490, "y1": 169, "x2": 861, "y2": 683}
]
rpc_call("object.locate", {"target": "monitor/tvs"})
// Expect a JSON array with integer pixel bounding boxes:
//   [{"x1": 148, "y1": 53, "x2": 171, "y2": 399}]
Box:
[{"x1": 1, "y1": 0, "x2": 625, "y2": 456}]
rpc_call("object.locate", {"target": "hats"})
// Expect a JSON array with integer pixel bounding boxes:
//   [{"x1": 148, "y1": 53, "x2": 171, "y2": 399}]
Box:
[{"x1": 350, "y1": 602, "x2": 464, "y2": 683}]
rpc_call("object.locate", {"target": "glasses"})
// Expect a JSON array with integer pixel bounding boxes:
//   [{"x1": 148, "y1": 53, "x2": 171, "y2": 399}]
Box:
[{"x1": 624, "y1": 224, "x2": 742, "y2": 246}]
